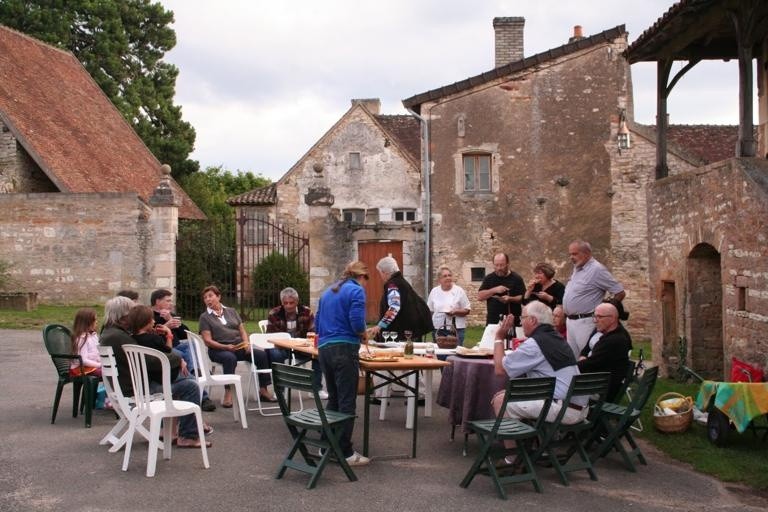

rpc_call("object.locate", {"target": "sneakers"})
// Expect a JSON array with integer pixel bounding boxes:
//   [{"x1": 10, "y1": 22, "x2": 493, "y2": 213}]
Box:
[
  {"x1": 203, "y1": 398, "x2": 233, "y2": 412},
  {"x1": 537, "y1": 453, "x2": 552, "y2": 467},
  {"x1": 318, "y1": 449, "x2": 370, "y2": 466},
  {"x1": 480, "y1": 458, "x2": 524, "y2": 475},
  {"x1": 308, "y1": 391, "x2": 329, "y2": 399}
]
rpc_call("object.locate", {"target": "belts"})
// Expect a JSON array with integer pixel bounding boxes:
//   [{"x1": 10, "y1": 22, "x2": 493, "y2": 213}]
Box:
[
  {"x1": 553, "y1": 398, "x2": 589, "y2": 410},
  {"x1": 567, "y1": 313, "x2": 594, "y2": 319}
]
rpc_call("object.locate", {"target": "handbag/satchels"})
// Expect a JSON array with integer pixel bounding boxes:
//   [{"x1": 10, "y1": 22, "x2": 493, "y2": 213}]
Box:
[
  {"x1": 358, "y1": 376, "x2": 373, "y2": 395},
  {"x1": 730, "y1": 358, "x2": 763, "y2": 383}
]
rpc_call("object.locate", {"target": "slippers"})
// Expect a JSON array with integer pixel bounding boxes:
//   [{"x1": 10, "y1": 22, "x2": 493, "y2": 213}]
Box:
[{"x1": 173, "y1": 423, "x2": 213, "y2": 447}]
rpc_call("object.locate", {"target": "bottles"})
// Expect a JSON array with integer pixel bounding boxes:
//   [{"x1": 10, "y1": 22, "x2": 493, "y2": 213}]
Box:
[
  {"x1": 499, "y1": 314, "x2": 515, "y2": 349},
  {"x1": 403, "y1": 330, "x2": 414, "y2": 360}
]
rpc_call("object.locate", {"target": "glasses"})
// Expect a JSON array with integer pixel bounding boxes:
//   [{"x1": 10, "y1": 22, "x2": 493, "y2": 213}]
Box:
[
  {"x1": 596, "y1": 315, "x2": 613, "y2": 320},
  {"x1": 520, "y1": 316, "x2": 530, "y2": 321}
]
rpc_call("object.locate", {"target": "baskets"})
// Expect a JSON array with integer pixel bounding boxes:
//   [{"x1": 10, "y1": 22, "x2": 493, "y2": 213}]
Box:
[
  {"x1": 436, "y1": 325, "x2": 458, "y2": 349},
  {"x1": 653, "y1": 392, "x2": 693, "y2": 433}
]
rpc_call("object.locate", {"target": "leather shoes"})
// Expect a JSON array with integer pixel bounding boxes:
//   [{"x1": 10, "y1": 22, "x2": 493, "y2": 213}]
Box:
[
  {"x1": 260, "y1": 392, "x2": 277, "y2": 402},
  {"x1": 370, "y1": 397, "x2": 390, "y2": 405},
  {"x1": 404, "y1": 399, "x2": 425, "y2": 406}
]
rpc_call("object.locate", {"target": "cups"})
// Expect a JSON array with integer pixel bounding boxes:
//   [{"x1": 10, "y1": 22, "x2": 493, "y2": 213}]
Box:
[{"x1": 424, "y1": 345, "x2": 434, "y2": 359}]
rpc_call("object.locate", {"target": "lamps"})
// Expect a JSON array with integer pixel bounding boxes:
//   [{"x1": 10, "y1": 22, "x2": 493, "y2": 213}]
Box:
[{"x1": 616, "y1": 106, "x2": 631, "y2": 150}]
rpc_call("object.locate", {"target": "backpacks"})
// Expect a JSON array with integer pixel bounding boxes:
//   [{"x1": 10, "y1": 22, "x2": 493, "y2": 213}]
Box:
[{"x1": 401, "y1": 286, "x2": 433, "y2": 334}]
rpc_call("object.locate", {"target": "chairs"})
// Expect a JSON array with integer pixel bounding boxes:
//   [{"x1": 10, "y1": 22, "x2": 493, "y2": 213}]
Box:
[{"x1": 458, "y1": 365, "x2": 660, "y2": 502}]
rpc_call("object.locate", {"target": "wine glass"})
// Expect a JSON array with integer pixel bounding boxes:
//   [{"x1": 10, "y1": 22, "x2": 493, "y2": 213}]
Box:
[{"x1": 381, "y1": 331, "x2": 398, "y2": 347}]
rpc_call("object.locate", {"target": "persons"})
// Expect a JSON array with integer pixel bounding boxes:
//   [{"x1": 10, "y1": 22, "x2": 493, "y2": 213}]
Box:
[
  {"x1": 481, "y1": 301, "x2": 591, "y2": 476},
  {"x1": 426, "y1": 263, "x2": 470, "y2": 375},
  {"x1": 198, "y1": 286, "x2": 278, "y2": 407},
  {"x1": 563, "y1": 235, "x2": 626, "y2": 363},
  {"x1": 477, "y1": 250, "x2": 526, "y2": 341},
  {"x1": 70, "y1": 290, "x2": 216, "y2": 450},
  {"x1": 370, "y1": 257, "x2": 434, "y2": 407},
  {"x1": 552, "y1": 305, "x2": 567, "y2": 343},
  {"x1": 315, "y1": 258, "x2": 372, "y2": 468},
  {"x1": 577, "y1": 297, "x2": 634, "y2": 422},
  {"x1": 266, "y1": 287, "x2": 329, "y2": 399},
  {"x1": 521, "y1": 261, "x2": 564, "y2": 309}
]
